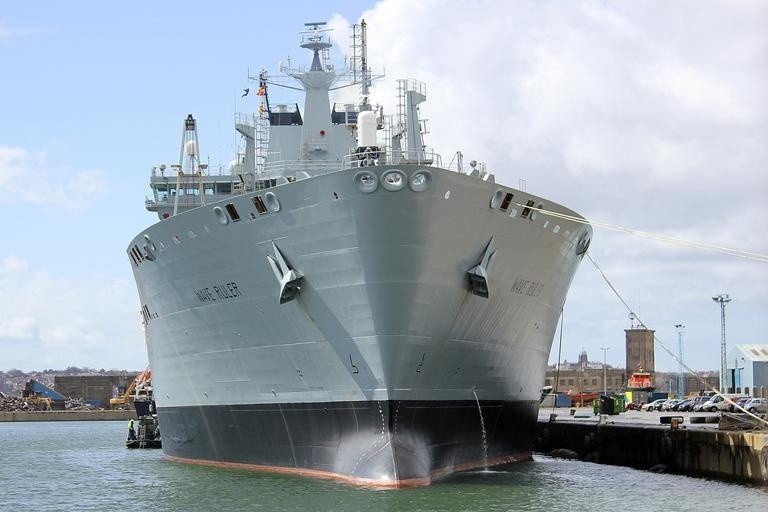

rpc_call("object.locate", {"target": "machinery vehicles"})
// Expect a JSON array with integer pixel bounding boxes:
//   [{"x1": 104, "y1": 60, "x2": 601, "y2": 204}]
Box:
[{"x1": 110, "y1": 368, "x2": 151, "y2": 409}]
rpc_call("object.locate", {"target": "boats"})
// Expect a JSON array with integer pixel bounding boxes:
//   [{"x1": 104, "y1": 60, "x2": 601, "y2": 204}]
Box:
[
  {"x1": 125, "y1": 415, "x2": 161, "y2": 448},
  {"x1": 129, "y1": 377, "x2": 157, "y2": 420}
]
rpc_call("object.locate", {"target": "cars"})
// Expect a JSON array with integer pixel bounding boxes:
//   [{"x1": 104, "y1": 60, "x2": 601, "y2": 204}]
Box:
[{"x1": 642, "y1": 394, "x2": 768, "y2": 413}]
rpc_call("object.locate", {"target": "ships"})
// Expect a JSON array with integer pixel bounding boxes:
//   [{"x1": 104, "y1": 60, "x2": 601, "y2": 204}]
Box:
[{"x1": 126, "y1": 19, "x2": 593, "y2": 489}]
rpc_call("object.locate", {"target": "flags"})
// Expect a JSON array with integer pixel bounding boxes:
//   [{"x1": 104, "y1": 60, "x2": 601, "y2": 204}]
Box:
[{"x1": 241, "y1": 88, "x2": 249, "y2": 98}]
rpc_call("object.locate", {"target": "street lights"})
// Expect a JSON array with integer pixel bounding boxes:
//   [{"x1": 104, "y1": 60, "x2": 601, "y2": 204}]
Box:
[
  {"x1": 672, "y1": 320, "x2": 689, "y2": 400},
  {"x1": 600, "y1": 344, "x2": 611, "y2": 396},
  {"x1": 712, "y1": 292, "x2": 734, "y2": 394}
]
